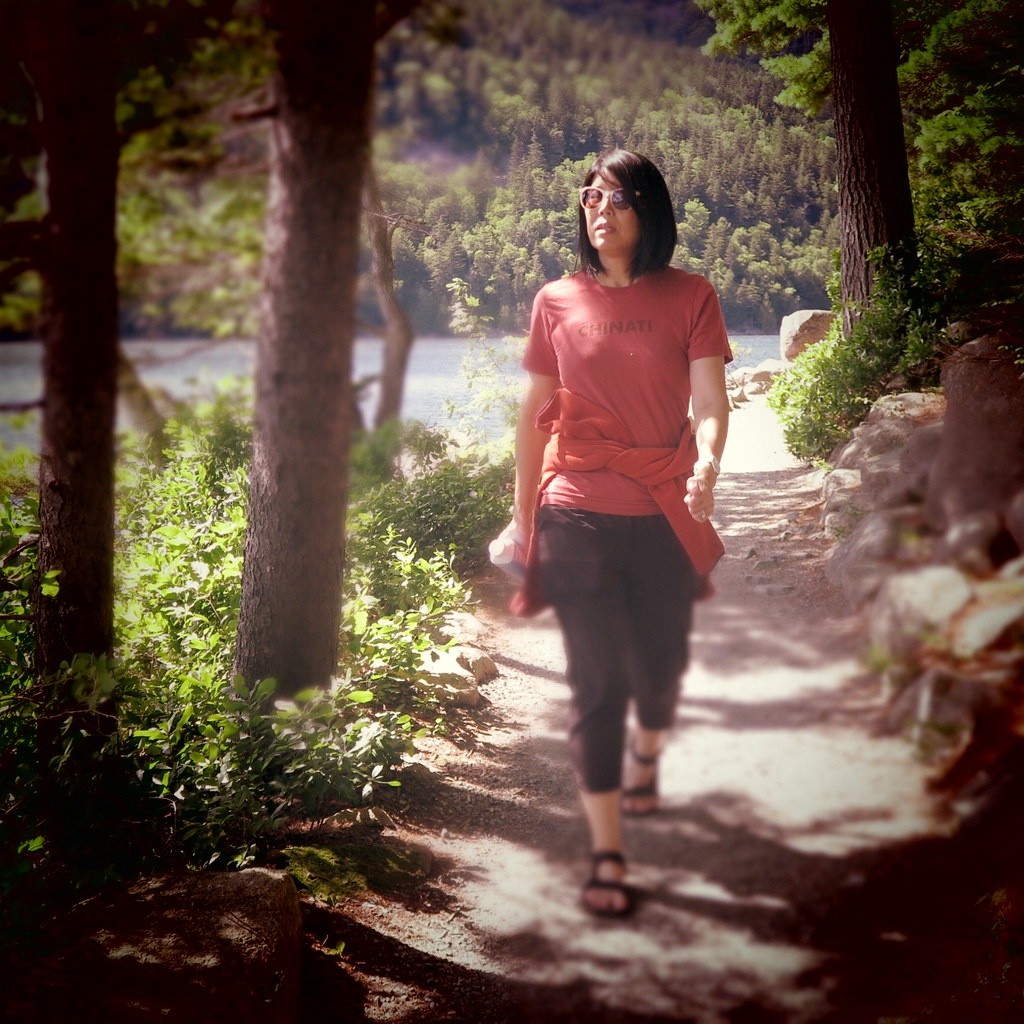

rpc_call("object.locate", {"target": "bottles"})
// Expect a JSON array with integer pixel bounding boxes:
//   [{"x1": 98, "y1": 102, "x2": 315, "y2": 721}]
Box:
[{"x1": 488, "y1": 539, "x2": 525, "y2": 583}]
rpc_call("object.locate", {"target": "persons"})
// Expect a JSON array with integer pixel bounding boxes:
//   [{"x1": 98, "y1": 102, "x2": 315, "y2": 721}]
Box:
[{"x1": 486, "y1": 147, "x2": 734, "y2": 917}]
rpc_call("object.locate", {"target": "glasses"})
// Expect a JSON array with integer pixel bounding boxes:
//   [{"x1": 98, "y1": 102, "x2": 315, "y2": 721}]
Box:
[{"x1": 578, "y1": 186, "x2": 633, "y2": 212}]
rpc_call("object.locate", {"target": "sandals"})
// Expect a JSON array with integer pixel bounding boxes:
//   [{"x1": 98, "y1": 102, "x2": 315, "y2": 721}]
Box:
[
  {"x1": 622, "y1": 729, "x2": 659, "y2": 817},
  {"x1": 580, "y1": 851, "x2": 630, "y2": 918}
]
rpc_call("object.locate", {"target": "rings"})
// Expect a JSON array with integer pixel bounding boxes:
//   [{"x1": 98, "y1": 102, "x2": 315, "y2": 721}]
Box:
[{"x1": 701, "y1": 511, "x2": 707, "y2": 518}]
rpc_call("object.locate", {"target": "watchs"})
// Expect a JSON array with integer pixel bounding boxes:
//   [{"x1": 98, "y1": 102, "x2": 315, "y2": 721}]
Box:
[{"x1": 694, "y1": 455, "x2": 721, "y2": 475}]
